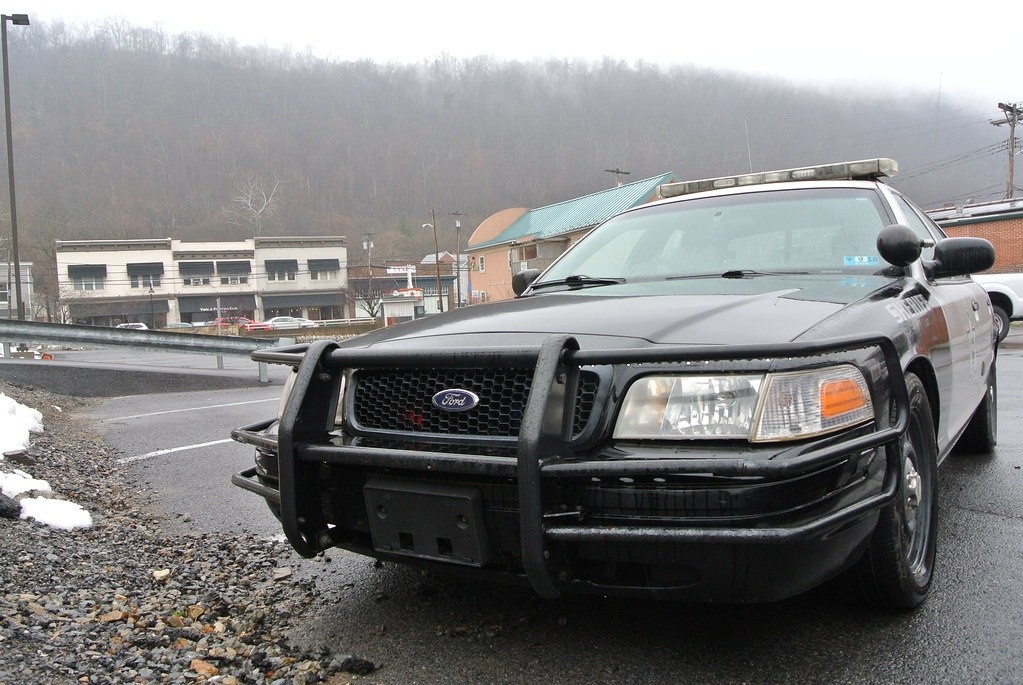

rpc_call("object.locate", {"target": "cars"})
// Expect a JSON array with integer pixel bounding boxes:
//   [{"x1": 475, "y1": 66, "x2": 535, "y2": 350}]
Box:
[
  {"x1": 113, "y1": 316, "x2": 321, "y2": 337},
  {"x1": 226, "y1": 155, "x2": 1001, "y2": 613},
  {"x1": 966, "y1": 271, "x2": 1023, "y2": 344}
]
